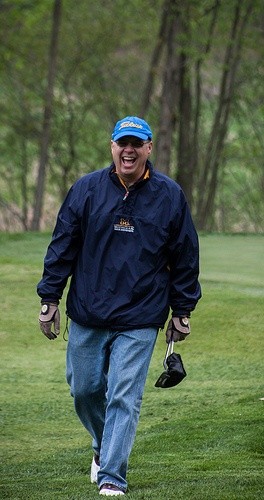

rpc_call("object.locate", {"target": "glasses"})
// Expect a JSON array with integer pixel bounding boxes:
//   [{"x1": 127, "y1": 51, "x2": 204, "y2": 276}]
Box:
[{"x1": 113, "y1": 139, "x2": 150, "y2": 148}]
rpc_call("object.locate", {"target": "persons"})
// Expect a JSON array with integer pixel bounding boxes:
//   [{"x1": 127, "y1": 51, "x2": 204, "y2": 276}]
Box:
[{"x1": 36, "y1": 116, "x2": 202, "y2": 497}]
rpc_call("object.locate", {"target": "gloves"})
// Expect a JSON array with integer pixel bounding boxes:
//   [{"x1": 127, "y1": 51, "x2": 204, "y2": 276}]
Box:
[
  {"x1": 166, "y1": 316, "x2": 190, "y2": 344},
  {"x1": 39, "y1": 302, "x2": 60, "y2": 340}
]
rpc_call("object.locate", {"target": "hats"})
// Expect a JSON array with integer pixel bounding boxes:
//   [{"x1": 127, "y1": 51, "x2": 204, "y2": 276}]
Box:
[{"x1": 111, "y1": 116, "x2": 152, "y2": 142}]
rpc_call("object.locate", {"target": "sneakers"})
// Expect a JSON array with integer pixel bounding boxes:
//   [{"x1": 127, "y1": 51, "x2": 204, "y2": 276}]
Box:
[
  {"x1": 99, "y1": 483, "x2": 126, "y2": 497},
  {"x1": 91, "y1": 454, "x2": 100, "y2": 483}
]
